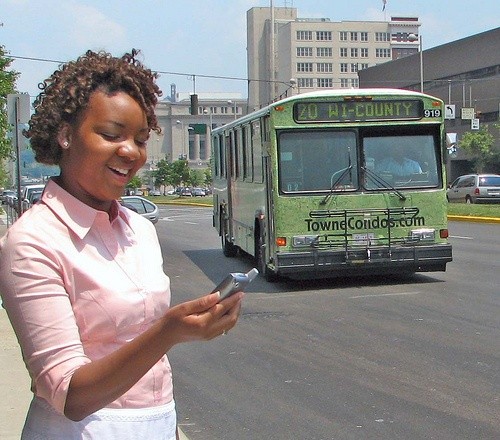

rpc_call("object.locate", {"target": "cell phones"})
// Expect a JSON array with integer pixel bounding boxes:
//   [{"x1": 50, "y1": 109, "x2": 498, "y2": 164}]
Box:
[{"x1": 206, "y1": 268, "x2": 264, "y2": 301}]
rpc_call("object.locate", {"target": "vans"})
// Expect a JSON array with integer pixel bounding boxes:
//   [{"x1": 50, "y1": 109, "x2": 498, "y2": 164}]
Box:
[
  {"x1": 445, "y1": 173, "x2": 500, "y2": 205},
  {"x1": 21, "y1": 184, "x2": 45, "y2": 212}
]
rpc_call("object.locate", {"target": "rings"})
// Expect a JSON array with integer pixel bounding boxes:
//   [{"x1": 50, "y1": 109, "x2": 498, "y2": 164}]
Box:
[{"x1": 223, "y1": 329, "x2": 226, "y2": 336}]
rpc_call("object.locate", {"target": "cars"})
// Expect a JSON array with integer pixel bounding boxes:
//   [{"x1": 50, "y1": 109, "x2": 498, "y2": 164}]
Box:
[
  {"x1": 0, "y1": 189, "x2": 15, "y2": 205},
  {"x1": 148, "y1": 190, "x2": 160, "y2": 196},
  {"x1": 23, "y1": 191, "x2": 44, "y2": 211},
  {"x1": 167, "y1": 186, "x2": 191, "y2": 197},
  {"x1": 119, "y1": 196, "x2": 159, "y2": 225},
  {"x1": 191, "y1": 188, "x2": 206, "y2": 197}
]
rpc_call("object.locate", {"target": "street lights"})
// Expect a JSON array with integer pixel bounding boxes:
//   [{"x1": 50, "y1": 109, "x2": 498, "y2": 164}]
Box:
[
  {"x1": 290, "y1": 78, "x2": 298, "y2": 96},
  {"x1": 406, "y1": 32, "x2": 424, "y2": 94},
  {"x1": 177, "y1": 120, "x2": 194, "y2": 158}
]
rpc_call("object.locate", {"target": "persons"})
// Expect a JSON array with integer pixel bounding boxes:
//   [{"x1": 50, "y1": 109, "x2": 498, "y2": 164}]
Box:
[
  {"x1": 0, "y1": 47, "x2": 244, "y2": 440},
  {"x1": 375, "y1": 142, "x2": 424, "y2": 175}
]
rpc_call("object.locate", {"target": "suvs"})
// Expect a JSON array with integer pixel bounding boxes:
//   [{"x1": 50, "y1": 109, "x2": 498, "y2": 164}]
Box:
[{"x1": 13, "y1": 189, "x2": 23, "y2": 214}]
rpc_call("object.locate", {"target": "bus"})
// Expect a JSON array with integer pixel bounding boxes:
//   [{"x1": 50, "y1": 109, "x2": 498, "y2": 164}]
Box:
[{"x1": 208, "y1": 86, "x2": 454, "y2": 283}]
